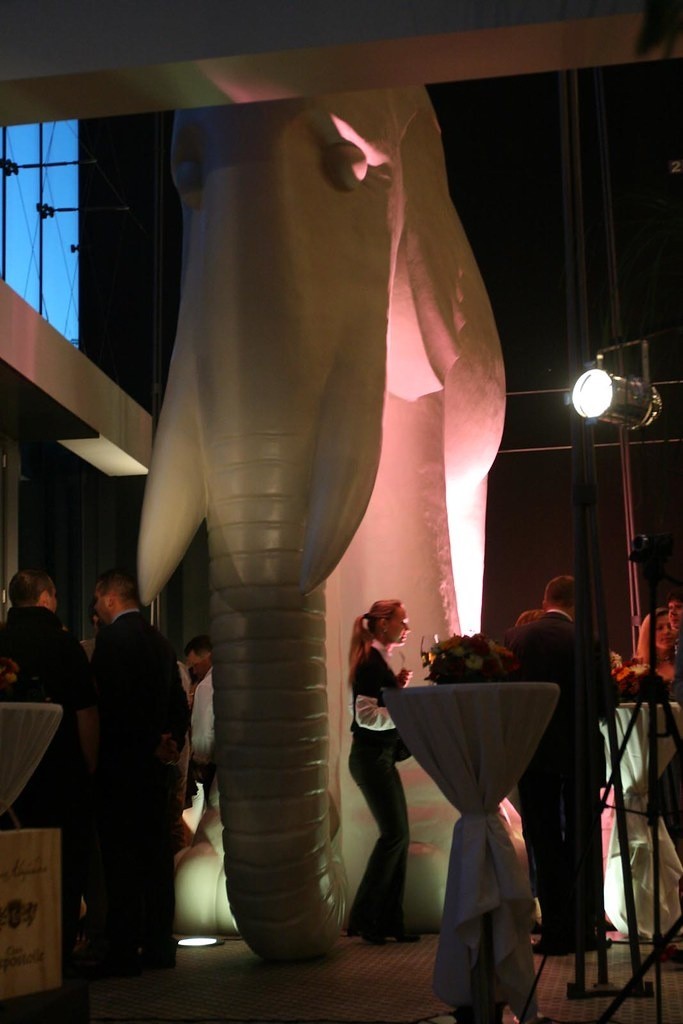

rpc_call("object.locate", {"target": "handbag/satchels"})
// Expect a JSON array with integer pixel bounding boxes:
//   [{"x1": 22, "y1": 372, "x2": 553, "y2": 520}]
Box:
[{"x1": 353, "y1": 694, "x2": 397, "y2": 731}]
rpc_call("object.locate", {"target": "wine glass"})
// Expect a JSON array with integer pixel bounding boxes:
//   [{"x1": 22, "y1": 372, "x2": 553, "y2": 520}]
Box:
[{"x1": 420, "y1": 634, "x2": 440, "y2": 685}]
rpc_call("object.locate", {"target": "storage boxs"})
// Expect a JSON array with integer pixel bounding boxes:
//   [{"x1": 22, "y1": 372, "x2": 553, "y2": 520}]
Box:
[{"x1": 0, "y1": 829, "x2": 64, "y2": 1000}]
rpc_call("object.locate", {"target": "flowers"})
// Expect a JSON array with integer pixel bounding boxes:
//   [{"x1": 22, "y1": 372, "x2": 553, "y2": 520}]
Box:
[
  {"x1": 420, "y1": 631, "x2": 524, "y2": 686},
  {"x1": 607, "y1": 657, "x2": 670, "y2": 703},
  {"x1": 0, "y1": 656, "x2": 21, "y2": 693}
]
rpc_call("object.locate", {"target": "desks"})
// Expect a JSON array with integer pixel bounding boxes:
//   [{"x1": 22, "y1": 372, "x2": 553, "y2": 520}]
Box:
[
  {"x1": 598, "y1": 703, "x2": 683, "y2": 942},
  {"x1": 0, "y1": 702, "x2": 65, "y2": 817},
  {"x1": 380, "y1": 681, "x2": 560, "y2": 1024}
]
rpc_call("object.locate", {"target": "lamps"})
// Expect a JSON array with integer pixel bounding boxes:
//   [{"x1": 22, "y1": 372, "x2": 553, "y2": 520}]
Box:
[
  {"x1": 565, "y1": 336, "x2": 665, "y2": 431},
  {"x1": 36, "y1": 202, "x2": 130, "y2": 220},
  {"x1": 4, "y1": 158, "x2": 98, "y2": 176}
]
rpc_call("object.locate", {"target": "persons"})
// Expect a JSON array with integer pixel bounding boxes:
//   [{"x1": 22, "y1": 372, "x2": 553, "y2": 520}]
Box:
[
  {"x1": 342, "y1": 599, "x2": 422, "y2": 945},
  {"x1": 636, "y1": 591, "x2": 683, "y2": 703},
  {"x1": 491, "y1": 576, "x2": 612, "y2": 956},
  {"x1": 0, "y1": 570, "x2": 215, "y2": 974}
]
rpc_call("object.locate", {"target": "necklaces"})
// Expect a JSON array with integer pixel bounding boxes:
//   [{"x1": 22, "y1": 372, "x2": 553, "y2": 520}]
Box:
[{"x1": 656, "y1": 656, "x2": 674, "y2": 663}]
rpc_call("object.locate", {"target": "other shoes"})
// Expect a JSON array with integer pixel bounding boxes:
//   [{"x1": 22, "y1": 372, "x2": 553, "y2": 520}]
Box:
[
  {"x1": 79, "y1": 953, "x2": 142, "y2": 977},
  {"x1": 346, "y1": 924, "x2": 387, "y2": 944},
  {"x1": 377, "y1": 927, "x2": 420, "y2": 944},
  {"x1": 576, "y1": 931, "x2": 612, "y2": 950},
  {"x1": 137, "y1": 948, "x2": 177, "y2": 969},
  {"x1": 531, "y1": 940, "x2": 568, "y2": 956}
]
